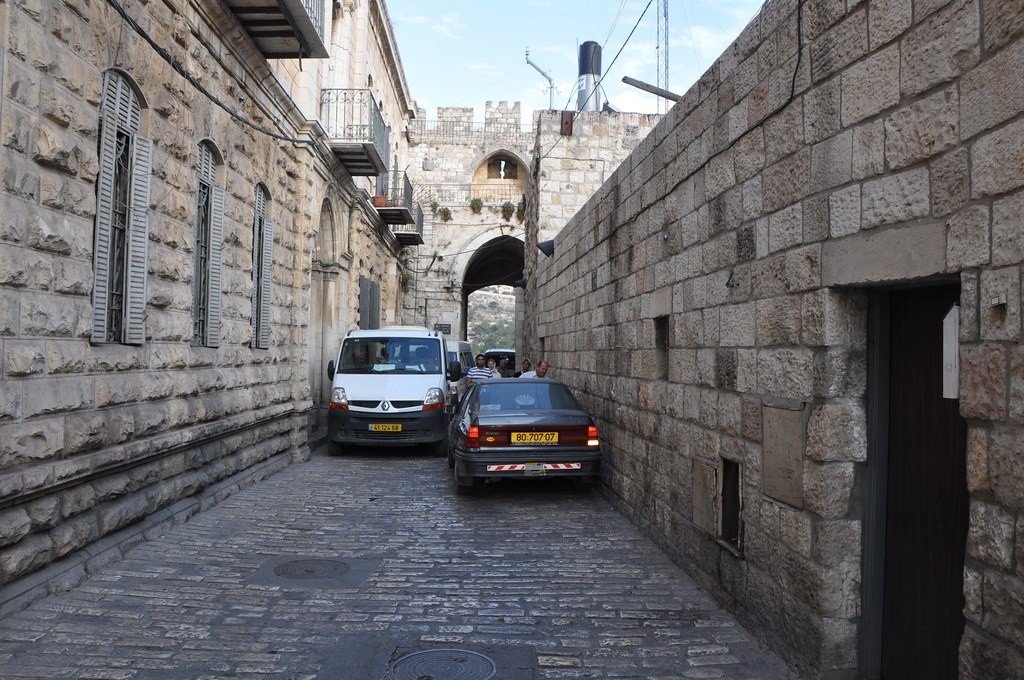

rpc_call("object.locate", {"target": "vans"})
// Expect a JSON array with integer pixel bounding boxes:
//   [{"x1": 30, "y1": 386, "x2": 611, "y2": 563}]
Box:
[
  {"x1": 480, "y1": 350, "x2": 517, "y2": 377},
  {"x1": 394, "y1": 340, "x2": 476, "y2": 415},
  {"x1": 328, "y1": 326, "x2": 460, "y2": 457}
]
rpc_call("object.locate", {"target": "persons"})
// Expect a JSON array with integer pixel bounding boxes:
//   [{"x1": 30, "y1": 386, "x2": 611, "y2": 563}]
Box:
[
  {"x1": 487, "y1": 359, "x2": 502, "y2": 378},
  {"x1": 519, "y1": 361, "x2": 549, "y2": 379},
  {"x1": 381, "y1": 348, "x2": 398, "y2": 364},
  {"x1": 496, "y1": 359, "x2": 510, "y2": 378},
  {"x1": 513, "y1": 358, "x2": 531, "y2": 378},
  {"x1": 466, "y1": 354, "x2": 493, "y2": 387}
]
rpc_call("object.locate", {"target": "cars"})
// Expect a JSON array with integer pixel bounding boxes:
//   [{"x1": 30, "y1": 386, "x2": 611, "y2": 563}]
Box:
[{"x1": 443, "y1": 377, "x2": 603, "y2": 495}]
